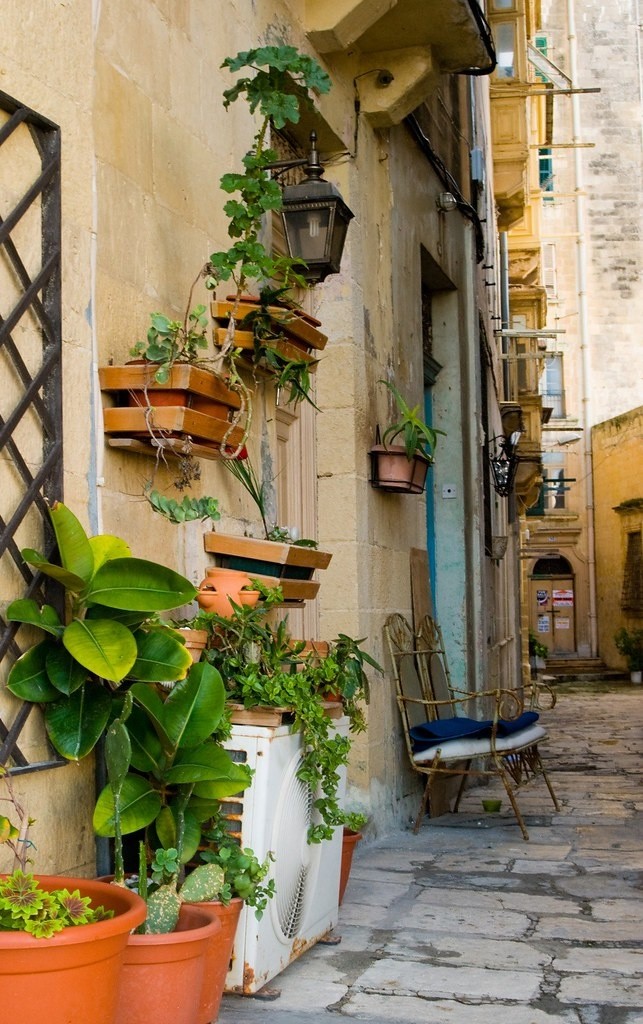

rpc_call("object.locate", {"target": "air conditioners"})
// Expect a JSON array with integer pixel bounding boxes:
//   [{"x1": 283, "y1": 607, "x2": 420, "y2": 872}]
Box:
[{"x1": 177, "y1": 717, "x2": 342, "y2": 1001}]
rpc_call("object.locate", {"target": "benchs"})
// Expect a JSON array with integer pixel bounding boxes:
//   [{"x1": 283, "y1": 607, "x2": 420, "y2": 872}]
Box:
[{"x1": 383, "y1": 611, "x2": 560, "y2": 844}]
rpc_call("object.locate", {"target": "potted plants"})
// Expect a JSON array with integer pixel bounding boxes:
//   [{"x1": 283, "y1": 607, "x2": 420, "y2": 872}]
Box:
[
  {"x1": 614, "y1": 627, "x2": 643, "y2": 683},
  {"x1": 0, "y1": 47, "x2": 386, "y2": 1024},
  {"x1": 371, "y1": 379, "x2": 448, "y2": 496}
]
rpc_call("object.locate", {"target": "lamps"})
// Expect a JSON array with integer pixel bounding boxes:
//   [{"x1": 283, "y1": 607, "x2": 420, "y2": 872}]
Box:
[
  {"x1": 260, "y1": 131, "x2": 355, "y2": 287},
  {"x1": 490, "y1": 431, "x2": 524, "y2": 498}
]
